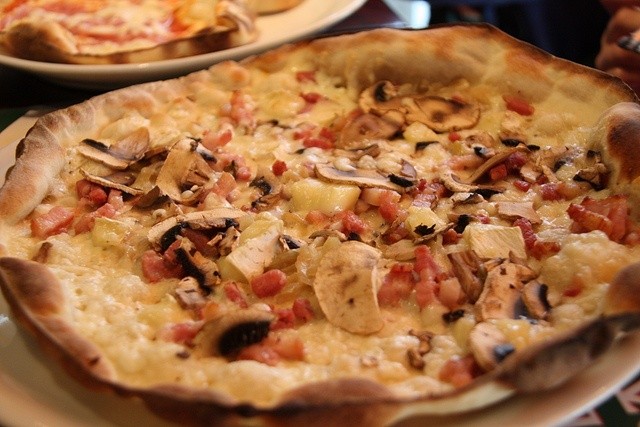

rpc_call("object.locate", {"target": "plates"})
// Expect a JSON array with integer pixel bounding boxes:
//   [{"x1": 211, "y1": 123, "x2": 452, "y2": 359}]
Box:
[{"x1": 0, "y1": 1, "x2": 366, "y2": 79}]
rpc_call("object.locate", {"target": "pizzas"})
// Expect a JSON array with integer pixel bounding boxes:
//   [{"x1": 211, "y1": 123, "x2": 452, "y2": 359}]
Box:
[{"x1": 0, "y1": 1, "x2": 303, "y2": 63}]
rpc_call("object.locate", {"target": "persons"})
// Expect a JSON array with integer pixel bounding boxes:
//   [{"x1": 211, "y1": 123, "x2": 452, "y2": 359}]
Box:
[{"x1": 594, "y1": 0, "x2": 640, "y2": 97}]
